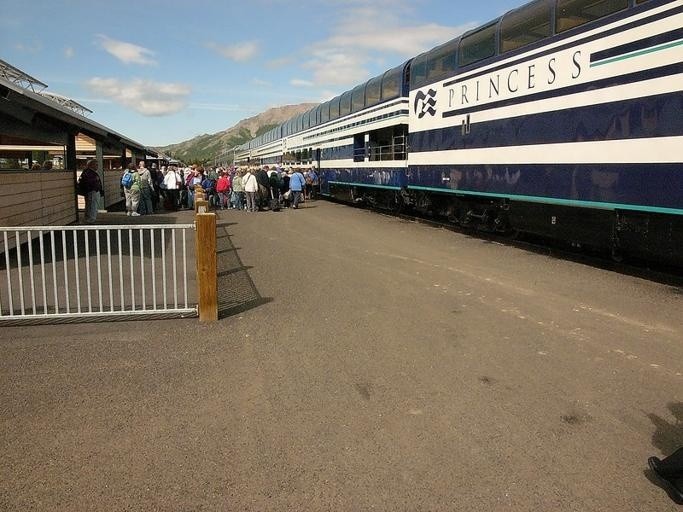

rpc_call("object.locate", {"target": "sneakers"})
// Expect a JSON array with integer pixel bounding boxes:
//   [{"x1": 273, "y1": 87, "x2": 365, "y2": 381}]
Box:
[{"x1": 127, "y1": 211, "x2": 141, "y2": 217}]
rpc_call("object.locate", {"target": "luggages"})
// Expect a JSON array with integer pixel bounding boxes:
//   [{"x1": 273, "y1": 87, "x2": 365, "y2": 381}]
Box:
[{"x1": 269, "y1": 198, "x2": 280, "y2": 212}]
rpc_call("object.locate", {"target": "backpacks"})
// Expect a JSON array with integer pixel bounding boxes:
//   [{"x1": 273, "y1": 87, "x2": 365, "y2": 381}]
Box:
[
  {"x1": 121, "y1": 169, "x2": 137, "y2": 189},
  {"x1": 76, "y1": 169, "x2": 87, "y2": 196}
]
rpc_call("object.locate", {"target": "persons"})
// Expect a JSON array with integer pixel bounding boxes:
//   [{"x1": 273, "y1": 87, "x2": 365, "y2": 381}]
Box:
[
  {"x1": 121, "y1": 158, "x2": 319, "y2": 219},
  {"x1": 648, "y1": 444, "x2": 683, "y2": 504},
  {"x1": 77, "y1": 158, "x2": 104, "y2": 225}
]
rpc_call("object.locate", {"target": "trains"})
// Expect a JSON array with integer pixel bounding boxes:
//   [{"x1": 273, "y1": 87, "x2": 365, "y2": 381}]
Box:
[{"x1": 211, "y1": 2, "x2": 682, "y2": 290}]
